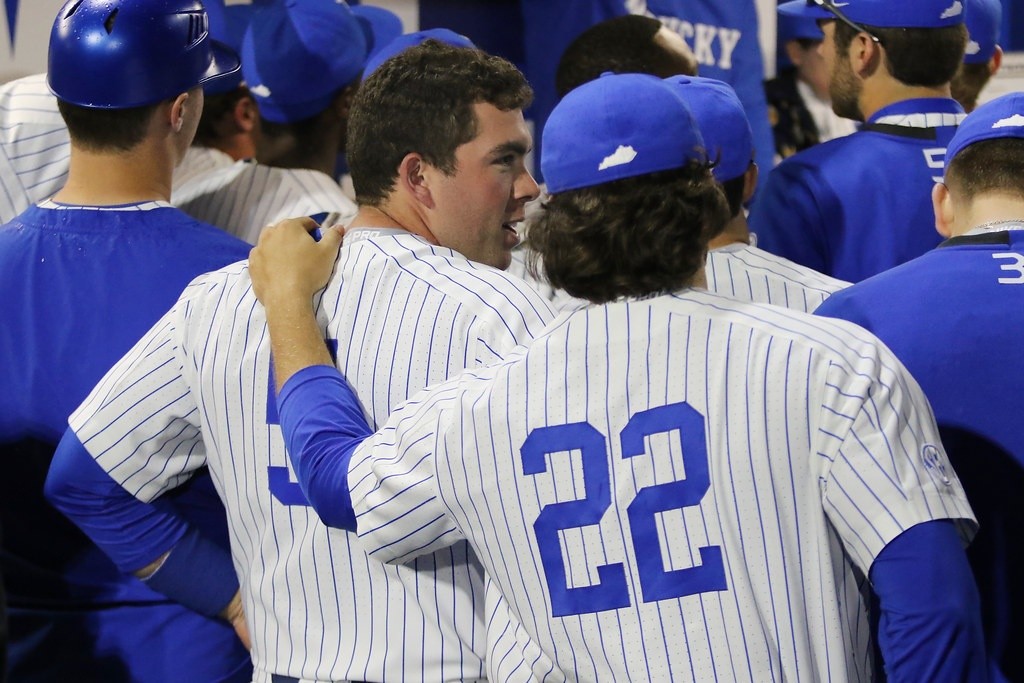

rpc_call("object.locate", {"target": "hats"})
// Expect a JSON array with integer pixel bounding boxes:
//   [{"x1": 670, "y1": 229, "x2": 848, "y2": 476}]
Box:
[
  {"x1": 943, "y1": 91, "x2": 1024, "y2": 181},
  {"x1": 775, "y1": 0, "x2": 965, "y2": 39},
  {"x1": 964, "y1": 1, "x2": 1002, "y2": 64},
  {"x1": 241, "y1": 0, "x2": 405, "y2": 123},
  {"x1": 665, "y1": 76, "x2": 754, "y2": 184},
  {"x1": 540, "y1": 71, "x2": 706, "y2": 194},
  {"x1": 361, "y1": 27, "x2": 478, "y2": 85}
]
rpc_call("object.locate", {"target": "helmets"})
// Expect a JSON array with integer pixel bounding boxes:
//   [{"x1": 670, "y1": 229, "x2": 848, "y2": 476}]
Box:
[{"x1": 45, "y1": 0, "x2": 242, "y2": 109}]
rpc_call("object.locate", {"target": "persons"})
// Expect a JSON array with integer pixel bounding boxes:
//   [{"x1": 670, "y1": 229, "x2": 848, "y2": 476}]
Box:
[
  {"x1": 0, "y1": 0, "x2": 259, "y2": 683},
  {"x1": 246, "y1": 69, "x2": 1009, "y2": 683},
  {"x1": 0, "y1": 0, "x2": 1024, "y2": 320},
  {"x1": 808, "y1": 86, "x2": 1024, "y2": 683},
  {"x1": 42, "y1": 35, "x2": 560, "y2": 683}
]
